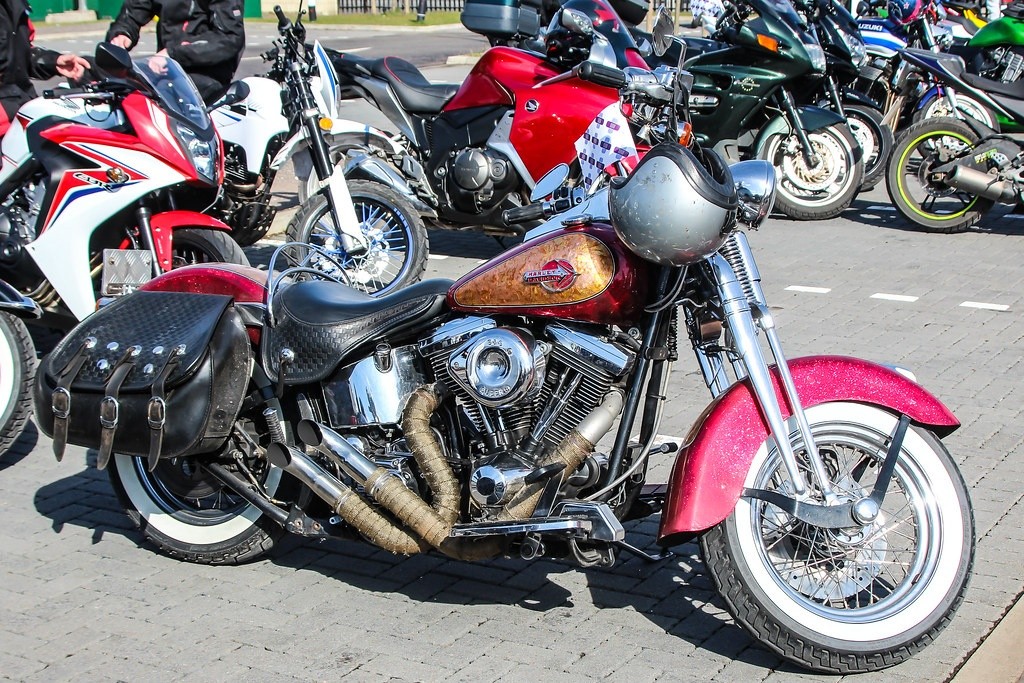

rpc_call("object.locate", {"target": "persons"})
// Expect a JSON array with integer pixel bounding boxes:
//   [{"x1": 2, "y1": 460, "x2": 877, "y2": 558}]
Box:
[
  {"x1": 0, "y1": 0, "x2": 91, "y2": 124},
  {"x1": 105, "y1": 0, "x2": 245, "y2": 107}
]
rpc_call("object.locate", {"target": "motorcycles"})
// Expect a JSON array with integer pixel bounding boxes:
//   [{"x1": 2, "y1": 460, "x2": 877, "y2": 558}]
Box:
[
  {"x1": 459, "y1": 0, "x2": 862, "y2": 219},
  {"x1": 0, "y1": 42, "x2": 250, "y2": 458},
  {"x1": 207, "y1": 4, "x2": 429, "y2": 295},
  {"x1": 886, "y1": 47, "x2": 1023, "y2": 231},
  {"x1": 609, "y1": 0, "x2": 1024, "y2": 190},
  {"x1": 33, "y1": 8, "x2": 975, "y2": 673},
  {"x1": 298, "y1": 11, "x2": 694, "y2": 245}
]
